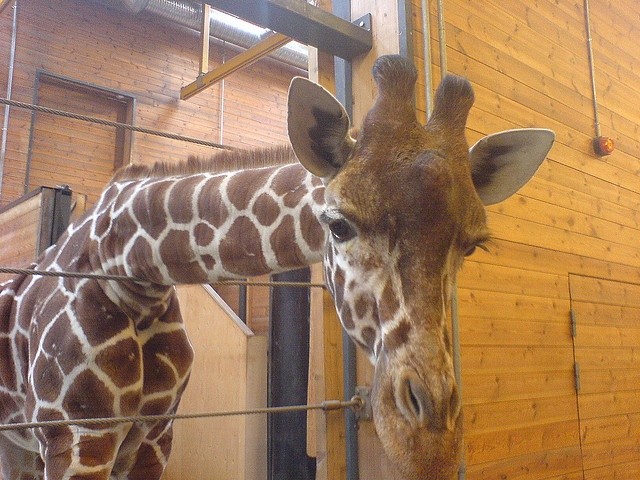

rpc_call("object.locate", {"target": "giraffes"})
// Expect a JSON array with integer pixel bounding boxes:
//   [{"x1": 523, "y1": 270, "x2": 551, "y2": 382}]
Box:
[{"x1": 0, "y1": 54, "x2": 556, "y2": 480}]
